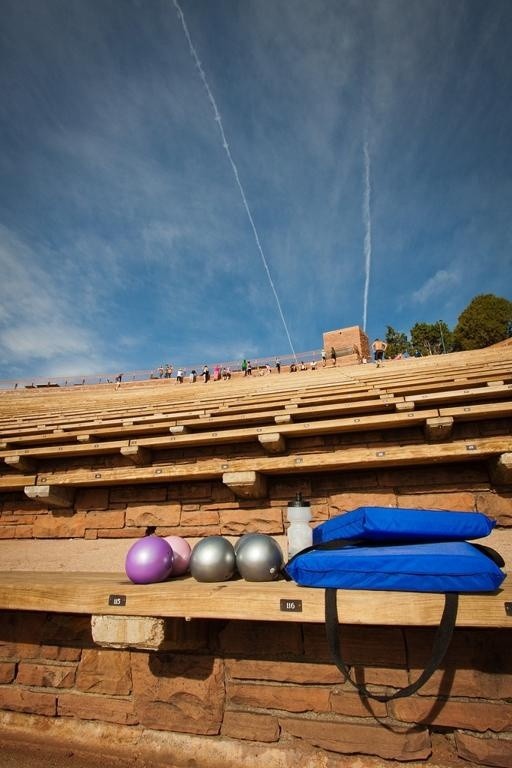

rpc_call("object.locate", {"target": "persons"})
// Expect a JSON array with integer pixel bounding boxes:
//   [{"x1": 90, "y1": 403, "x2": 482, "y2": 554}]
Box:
[
  {"x1": 113, "y1": 373, "x2": 123, "y2": 391},
  {"x1": 158, "y1": 338, "x2": 422, "y2": 385}
]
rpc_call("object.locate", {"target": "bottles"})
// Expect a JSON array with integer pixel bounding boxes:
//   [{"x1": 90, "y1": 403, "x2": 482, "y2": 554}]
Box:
[{"x1": 286, "y1": 491, "x2": 315, "y2": 560}]
